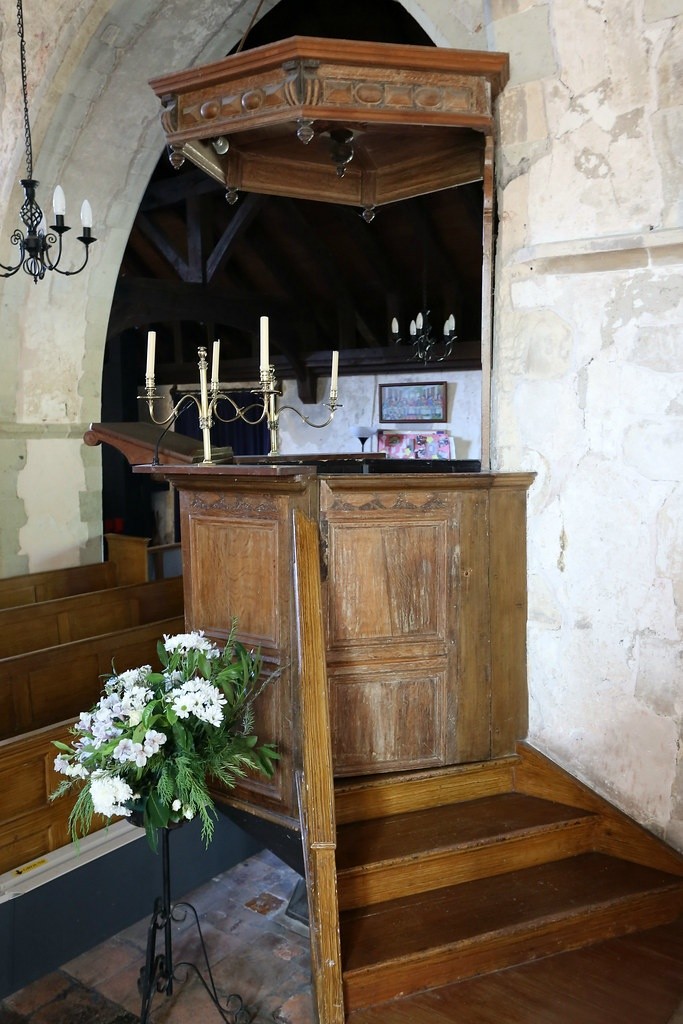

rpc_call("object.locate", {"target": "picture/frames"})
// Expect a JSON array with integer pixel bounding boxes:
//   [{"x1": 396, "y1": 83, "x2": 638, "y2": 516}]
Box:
[{"x1": 378, "y1": 381, "x2": 447, "y2": 423}]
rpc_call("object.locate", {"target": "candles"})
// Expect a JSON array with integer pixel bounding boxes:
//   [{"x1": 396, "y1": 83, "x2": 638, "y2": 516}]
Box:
[
  {"x1": 331, "y1": 351, "x2": 338, "y2": 390},
  {"x1": 260, "y1": 317, "x2": 269, "y2": 371},
  {"x1": 211, "y1": 339, "x2": 219, "y2": 381},
  {"x1": 146, "y1": 331, "x2": 156, "y2": 378}
]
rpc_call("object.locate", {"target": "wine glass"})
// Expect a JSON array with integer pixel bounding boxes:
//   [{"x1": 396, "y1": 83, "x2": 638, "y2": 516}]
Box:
[{"x1": 357, "y1": 427, "x2": 370, "y2": 453}]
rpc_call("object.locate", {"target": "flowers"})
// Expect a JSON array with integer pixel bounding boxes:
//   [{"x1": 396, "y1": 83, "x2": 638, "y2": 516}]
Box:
[{"x1": 54, "y1": 625, "x2": 281, "y2": 842}]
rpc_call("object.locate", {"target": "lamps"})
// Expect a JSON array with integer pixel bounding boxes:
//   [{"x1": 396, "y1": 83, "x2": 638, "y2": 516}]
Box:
[
  {"x1": 0, "y1": 0, "x2": 98, "y2": 284},
  {"x1": 386, "y1": 273, "x2": 457, "y2": 365}
]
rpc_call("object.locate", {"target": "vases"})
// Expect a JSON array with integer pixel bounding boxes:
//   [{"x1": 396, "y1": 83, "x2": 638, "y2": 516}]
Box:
[{"x1": 121, "y1": 780, "x2": 196, "y2": 828}]
